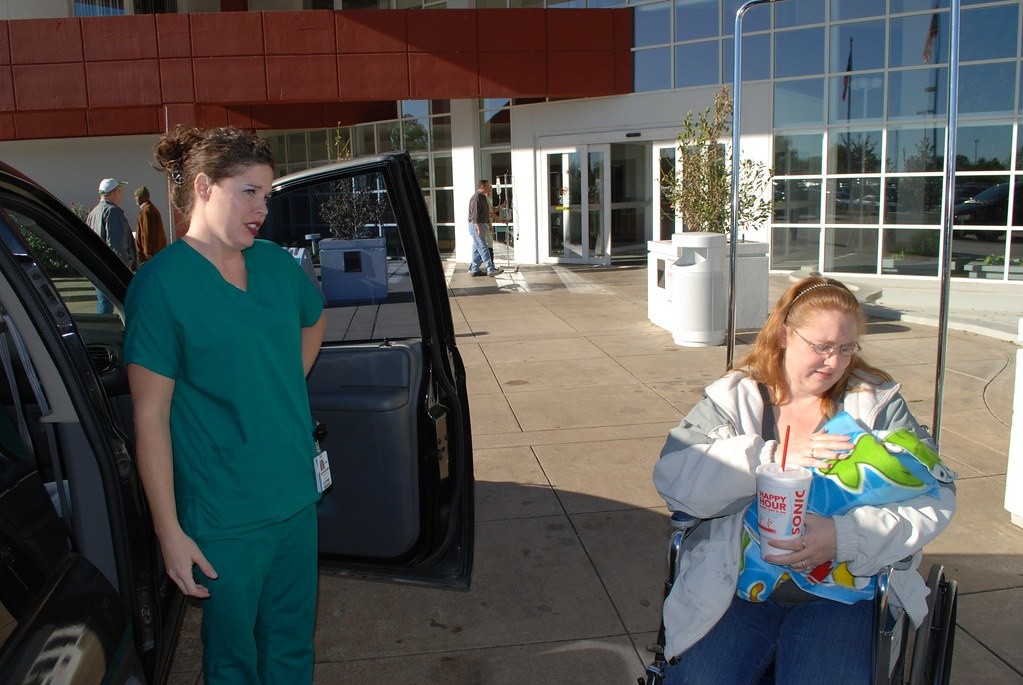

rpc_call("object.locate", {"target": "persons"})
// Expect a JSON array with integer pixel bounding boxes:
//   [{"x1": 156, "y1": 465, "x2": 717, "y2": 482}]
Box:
[
  {"x1": 468, "y1": 180, "x2": 509, "y2": 277},
  {"x1": 134, "y1": 186, "x2": 166, "y2": 266},
  {"x1": 85, "y1": 178, "x2": 135, "y2": 314},
  {"x1": 652, "y1": 276, "x2": 957, "y2": 685},
  {"x1": 122, "y1": 124, "x2": 326, "y2": 685},
  {"x1": 320, "y1": 461, "x2": 325, "y2": 470}
]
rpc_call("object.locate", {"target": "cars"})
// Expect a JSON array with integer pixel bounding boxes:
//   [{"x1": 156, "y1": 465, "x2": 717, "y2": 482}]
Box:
[
  {"x1": 935, "y1": 181, "x2": 1023, "y2": 240},
  {"x1": 773, "y1": 181, "x2": 993, "y2": 213},
  {"x1": 0, "y1": 149, "x2": 476, "y2": 685}
]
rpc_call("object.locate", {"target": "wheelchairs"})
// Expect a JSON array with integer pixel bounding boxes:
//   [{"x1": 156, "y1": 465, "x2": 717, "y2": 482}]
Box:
[{"x1": 640, "y1": 512, "x2": 959, "y2": 685}]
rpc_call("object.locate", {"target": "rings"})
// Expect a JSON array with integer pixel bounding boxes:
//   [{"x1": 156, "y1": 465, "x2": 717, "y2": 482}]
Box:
[
  {"x1": 802, "y1": 561, "x2": 806, "y2": 567},
  {"x1": 810, "y1": 449, "x2": 814, "y2": 458},
  {"x1": 802, "y1": 539, "x2": 805, "y2": 548}
]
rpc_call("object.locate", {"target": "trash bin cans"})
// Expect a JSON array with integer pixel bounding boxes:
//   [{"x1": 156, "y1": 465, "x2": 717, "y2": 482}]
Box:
[{"x1": 671, "y1": 233, "x2": 727, "y2": 348}]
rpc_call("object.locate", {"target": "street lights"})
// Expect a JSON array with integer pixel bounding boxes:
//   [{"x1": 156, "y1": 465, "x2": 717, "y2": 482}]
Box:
[{"x1": 915, "y1": 87, "x2": 980, "y2": 166}]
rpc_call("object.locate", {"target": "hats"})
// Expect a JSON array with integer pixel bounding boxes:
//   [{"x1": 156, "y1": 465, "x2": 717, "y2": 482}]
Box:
[
  {"x1": 134, "y1": 185, "x2": 150, "y2": 200},
  {"x1": 98, "y1": 178, "x2": 128, "y2": 196}
]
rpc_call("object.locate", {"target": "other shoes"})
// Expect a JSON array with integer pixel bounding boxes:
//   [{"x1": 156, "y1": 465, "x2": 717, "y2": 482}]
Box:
[
  {"x1": 472, "y1": 270, "x2": 487, "y2": 276},
  {"x1": 489, "y1": 268, "x2": 504, "y2": 277}
]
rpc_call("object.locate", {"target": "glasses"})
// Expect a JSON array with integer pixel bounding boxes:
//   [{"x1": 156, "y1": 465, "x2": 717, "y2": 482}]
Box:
[{"x1": 789, "y1": 327, "x2": 862, "y2": 356}]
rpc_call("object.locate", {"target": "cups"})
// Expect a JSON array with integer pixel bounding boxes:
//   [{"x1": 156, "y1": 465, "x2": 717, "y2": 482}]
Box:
[{"x1": 756, "y1": 463, "x2": 813, "y2": 562}]
